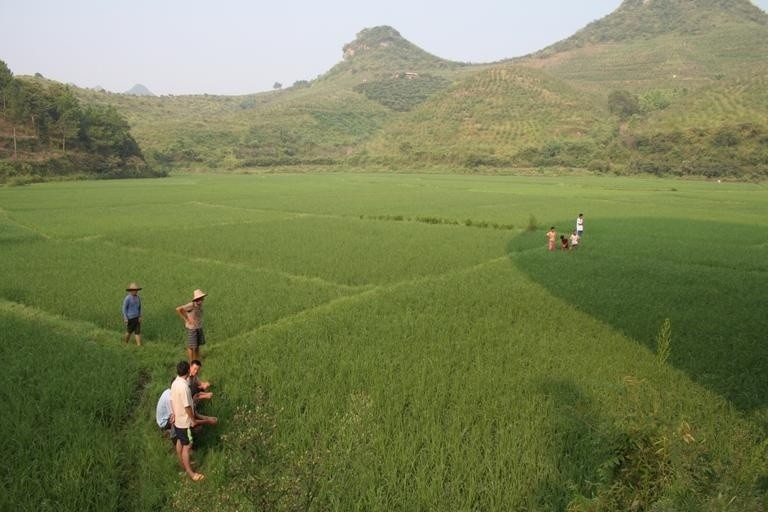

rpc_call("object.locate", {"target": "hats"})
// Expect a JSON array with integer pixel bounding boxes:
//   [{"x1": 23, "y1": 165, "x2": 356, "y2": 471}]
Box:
[
  {"x1": 125, "y1": 282, "x2": 142, "y2": 292},
  {"x1": 192, "y1": 288, "x2": 207, "y2": 302}
]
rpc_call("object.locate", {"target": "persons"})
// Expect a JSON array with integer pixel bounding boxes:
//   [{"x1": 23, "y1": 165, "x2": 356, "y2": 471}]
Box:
[
  {"x1": 576, "y1": 214, "x2": 584, "y2": 237},
  {"x1": 156, "y1": 358, "x2": 218, "y2": 481},
  {"x1": 546, "y1": 226, "x2": 556, "y2": 251},
  {"x1": 560, "y1": 235, "x2": 569, "y2": 251},
  {"x1": 175, "y1": 288, "x2": 208, "y2": 363},
  {"x1": 569, "y1": 229, "x2": 580, "y2": 248},
  {"x1": 122, "y1": 282, "x2": 143, "y2": 346}
]
